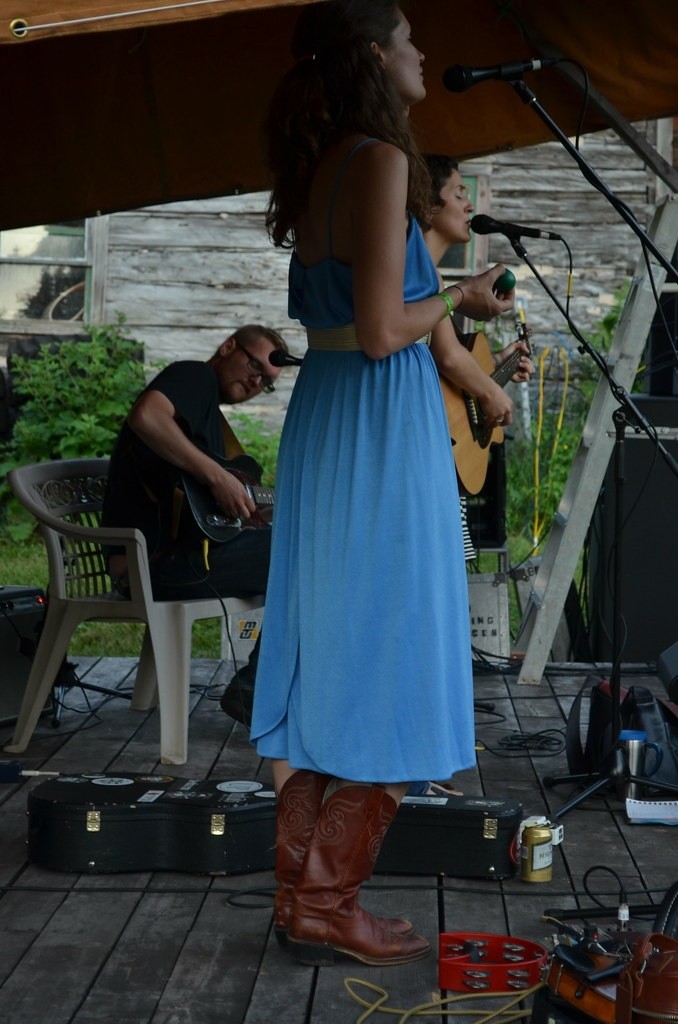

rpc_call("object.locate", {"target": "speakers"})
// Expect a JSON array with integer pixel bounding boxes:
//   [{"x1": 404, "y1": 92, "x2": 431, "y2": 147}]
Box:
[
  {"x1": 584, "y1": 391, "x2": 677, "y2": 670},
  {"x1": 0, "y1": 584, "x2": 59, "y2": 728}
]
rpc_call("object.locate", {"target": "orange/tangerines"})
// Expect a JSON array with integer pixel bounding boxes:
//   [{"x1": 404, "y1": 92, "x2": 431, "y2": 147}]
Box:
[{"x1": 495, "y1": 269, "x2": 516, "y2": 292}]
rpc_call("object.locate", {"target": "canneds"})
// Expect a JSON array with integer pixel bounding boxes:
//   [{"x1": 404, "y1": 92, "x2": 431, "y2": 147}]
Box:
[{"x1": 521, "y1": 826, "x2": 553, "y2": 884}]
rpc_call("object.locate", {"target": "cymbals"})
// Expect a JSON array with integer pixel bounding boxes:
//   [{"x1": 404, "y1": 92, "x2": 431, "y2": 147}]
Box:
[{"x1": 440, "y1": 927, "x2": 547, "y2": 998}]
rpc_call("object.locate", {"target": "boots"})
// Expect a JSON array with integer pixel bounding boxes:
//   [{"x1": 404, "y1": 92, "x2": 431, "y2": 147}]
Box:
[
  {"x1": 273, "y1": 767, "x2": 415, "y2": 952},
  {"x1": 284, "y1": 775, "x2": 430, "y2": 967}
]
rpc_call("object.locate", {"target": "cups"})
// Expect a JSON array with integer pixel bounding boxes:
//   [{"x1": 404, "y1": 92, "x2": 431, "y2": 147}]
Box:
[{"x1": 616, "y1": 729, "x2": 662, "y2": 802}]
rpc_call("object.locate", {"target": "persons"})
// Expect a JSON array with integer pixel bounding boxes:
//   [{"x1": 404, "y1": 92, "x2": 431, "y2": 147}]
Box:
[
  {"x1": 419, "y1": 149, "x2": 536, "y2": 564},
  {"x1": 250, "y1": 0, "x2": 516, "y2": 969},
  {"x1": 100, "y1": 323, "x2": 289, "y2": 729}
]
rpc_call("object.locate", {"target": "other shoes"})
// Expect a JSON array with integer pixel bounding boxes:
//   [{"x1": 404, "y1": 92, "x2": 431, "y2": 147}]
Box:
[{"x1": 220, "y1": 668, "x2": 255, "y2": 727}]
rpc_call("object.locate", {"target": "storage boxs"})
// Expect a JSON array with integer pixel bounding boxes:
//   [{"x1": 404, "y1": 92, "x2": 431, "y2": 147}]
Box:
[{"x1": 466, "y1": 573, "x2": 510, "y2": 663}]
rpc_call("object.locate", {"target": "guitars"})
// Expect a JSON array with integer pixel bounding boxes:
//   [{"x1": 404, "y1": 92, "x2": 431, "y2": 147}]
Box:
[
  {"x1": 173, "y1": 454, "x2": 276, "y2": 544},
  {"x1": 438, "y1": 330, "x2": 533, "y2": 499}
]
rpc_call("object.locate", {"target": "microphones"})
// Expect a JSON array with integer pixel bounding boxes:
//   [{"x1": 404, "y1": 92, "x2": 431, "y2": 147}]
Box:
[
  {"x1": 444, "y1": 54, "x2": 564, "y2": 93},
  {"x1": 268, "y1": 349, "x2": 303, "y2": 367},
  {"x1": 470, "y1": 214, "x2": 561, "y2": 241}
]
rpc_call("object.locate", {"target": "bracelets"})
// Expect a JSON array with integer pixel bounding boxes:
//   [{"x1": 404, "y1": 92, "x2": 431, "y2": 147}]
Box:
[{"x1": 435, "y1": 292, "x2": 454, "y2": 317}]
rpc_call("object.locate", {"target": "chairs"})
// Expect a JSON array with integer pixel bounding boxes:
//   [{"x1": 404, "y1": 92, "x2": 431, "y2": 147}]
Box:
[{"x1": 8, "y1": 458, "x2": 265, "y2": 765}]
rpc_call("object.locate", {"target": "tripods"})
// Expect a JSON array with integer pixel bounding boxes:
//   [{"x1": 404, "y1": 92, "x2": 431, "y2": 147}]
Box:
[{"x1": 505, "y1": 234, "x2": 677, "y2": 825}]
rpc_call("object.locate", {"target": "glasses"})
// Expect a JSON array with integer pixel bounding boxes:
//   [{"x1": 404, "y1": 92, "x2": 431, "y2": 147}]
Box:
[{"x1": 236, "y1": 343, "x2": 276, "y2": 394}]
